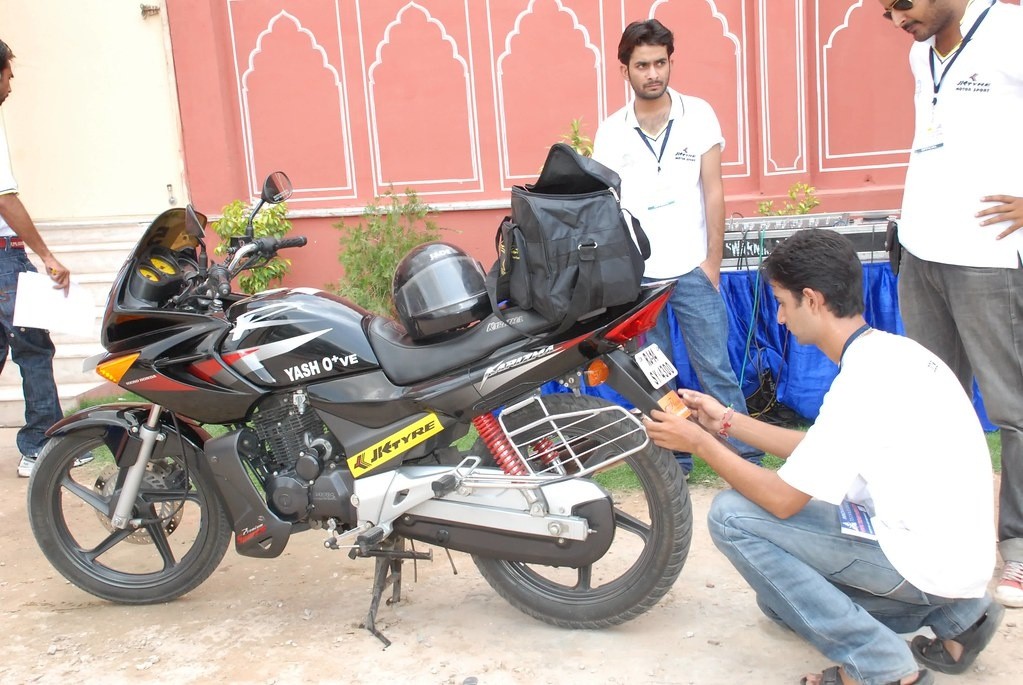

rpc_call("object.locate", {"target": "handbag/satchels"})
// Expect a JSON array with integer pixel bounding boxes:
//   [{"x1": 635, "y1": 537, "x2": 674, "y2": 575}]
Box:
[{"x1": 484, "y1": 143, "x2": 651, "y2": 339}]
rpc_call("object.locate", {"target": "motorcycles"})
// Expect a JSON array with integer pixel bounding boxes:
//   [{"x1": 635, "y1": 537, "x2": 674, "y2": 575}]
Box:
[{"x1": 26, "y1": 173, "x2": 692, "y2": 649}]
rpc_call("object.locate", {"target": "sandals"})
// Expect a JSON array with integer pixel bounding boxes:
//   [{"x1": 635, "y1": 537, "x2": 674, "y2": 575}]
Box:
[
  {"x1": 911, "y1": 599, "x2": 1005, "y2": 675},
  {"x1": 800, "y1": 661, "x2": 934, "y2": 685}
]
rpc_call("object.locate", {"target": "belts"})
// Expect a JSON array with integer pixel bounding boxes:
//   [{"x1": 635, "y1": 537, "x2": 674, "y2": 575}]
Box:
[{"x1": 0, "y1": 238, "x2": 24, "y2": 249}]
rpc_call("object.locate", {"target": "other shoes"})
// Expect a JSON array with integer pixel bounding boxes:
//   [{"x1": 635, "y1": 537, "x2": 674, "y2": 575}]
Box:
[
  {"x1": 679, "y1": 464, "x2": 690, "y2": 480},
  {"x1": 745, "y1": 456, "x2": 764, "y2": 468}
]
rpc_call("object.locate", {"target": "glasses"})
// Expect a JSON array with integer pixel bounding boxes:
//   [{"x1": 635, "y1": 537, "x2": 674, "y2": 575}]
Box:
[{"x1": 882, "y1": 0, "x2": 913, "y2": 20}]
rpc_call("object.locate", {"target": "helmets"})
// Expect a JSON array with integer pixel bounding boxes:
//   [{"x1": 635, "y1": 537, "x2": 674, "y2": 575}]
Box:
[{"x1": 393, "y1": 242, "x2": 493, "y2": 345}]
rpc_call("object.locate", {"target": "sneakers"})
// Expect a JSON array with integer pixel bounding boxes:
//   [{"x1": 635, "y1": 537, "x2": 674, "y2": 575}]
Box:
[
  {"x1": 17, "y1": 446, "x2": 95, "y2": 478},
  {"x1": 995, "y1": 561, "x2": 1023, "y2": 608}
]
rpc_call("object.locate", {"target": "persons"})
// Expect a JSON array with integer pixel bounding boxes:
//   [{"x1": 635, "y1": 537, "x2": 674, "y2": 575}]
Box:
[
  {"x1": 876, "y1": 0, "x2": 1023, "y2": 610},
  {"x1": 0, "y1": 37, "x2": 96, "y2": 480},
  {"x1": 641, "y1": 226, "x2": 1005, "y2": 685},
  {"x1": 590, "y1": 17, "x2": 767, "y2": 483}
]
